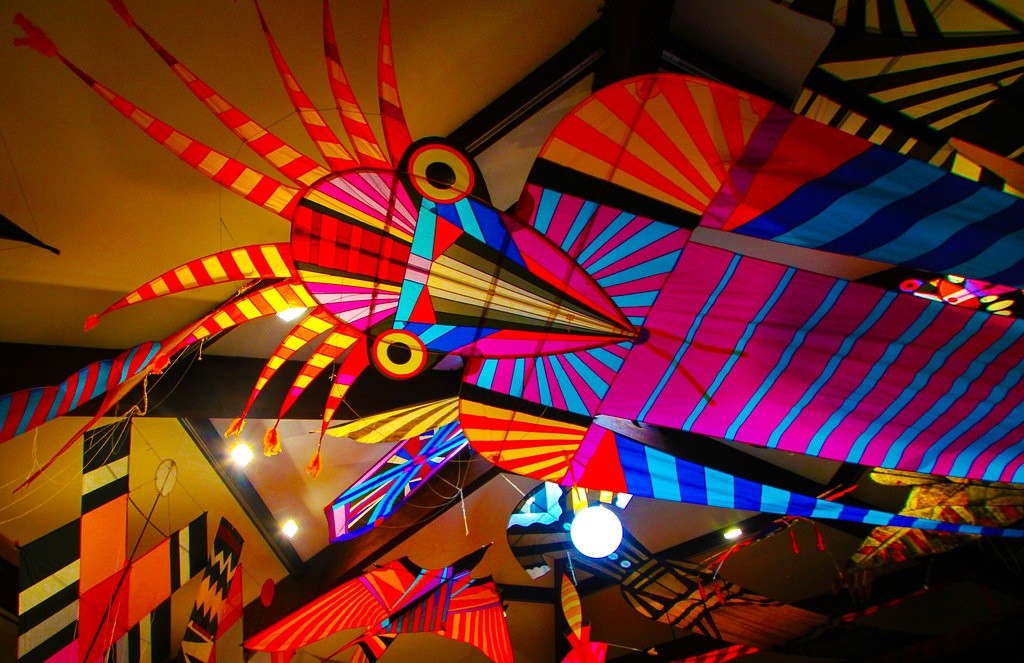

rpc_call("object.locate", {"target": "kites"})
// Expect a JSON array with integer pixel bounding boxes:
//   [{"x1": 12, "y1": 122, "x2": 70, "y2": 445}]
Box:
[
  {"x1": 178, "y1": 515, "x2": 245, "y2": 663},
  {"x1": 17, "y1": 0, "x2": 1024, "y2": 540},
  {"x1": 838, "y1": 469, "x2": 1023, "y2": 597},
  {"x1": 15, "y1": 413, "x2": 211, "y2": 663},
  {"x1": 236, "y1": 541, "x2": 513, "y2": 663},
  {"x1": 787, "y1": 1, "x2": 1023, "y2": 319},
  {"x1": 504, "y1": 478, "x2": 827, "y2": 648}
]
